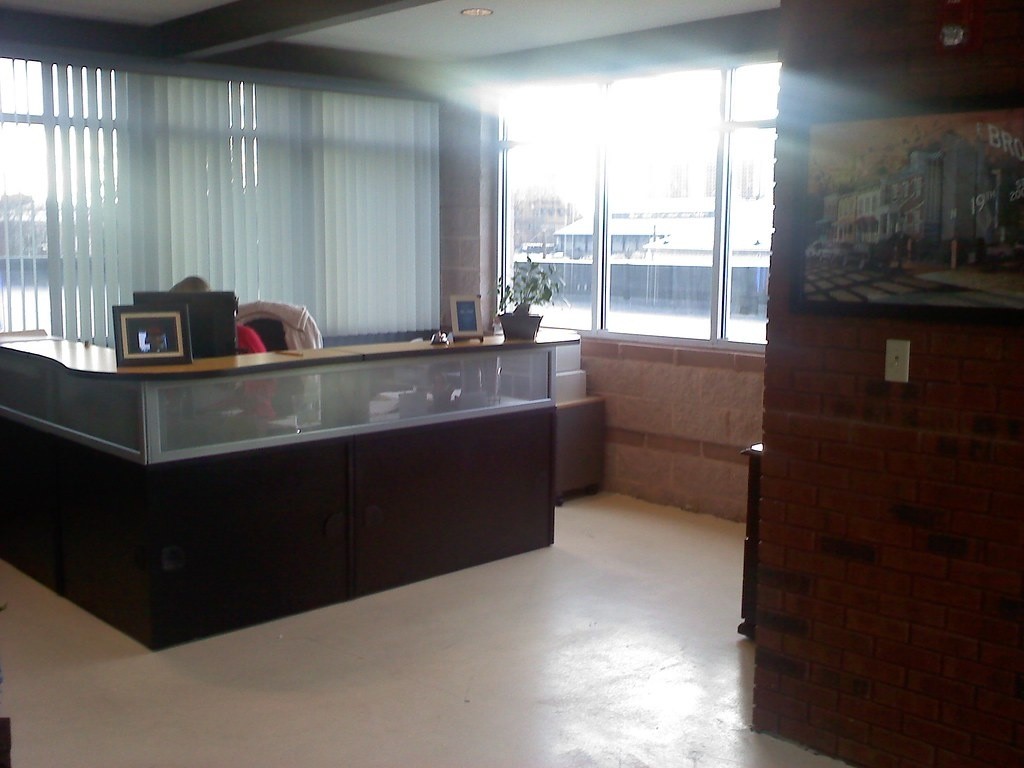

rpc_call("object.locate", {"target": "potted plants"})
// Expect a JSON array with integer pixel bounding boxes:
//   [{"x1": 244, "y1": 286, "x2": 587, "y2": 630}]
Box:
[{"x1": 494, "y1": 258, "x2": 572, "y2": 342}]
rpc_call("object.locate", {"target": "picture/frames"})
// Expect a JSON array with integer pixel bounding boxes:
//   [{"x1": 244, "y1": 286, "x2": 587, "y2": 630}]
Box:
[
  {"x1": 111, "y1": 303, "x2": 195, "y2": 370},
  {"x1": 450, "y1": 294, "x2": 483, "y2": 336},
  {"x1": 788, "y1": 87, "x2": 1023, "y2": 321}
]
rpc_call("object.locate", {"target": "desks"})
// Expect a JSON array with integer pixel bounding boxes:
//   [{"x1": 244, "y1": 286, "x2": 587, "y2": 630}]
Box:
[{"x1": 736, "y1": 439, "x2": 764, "y2": 642}]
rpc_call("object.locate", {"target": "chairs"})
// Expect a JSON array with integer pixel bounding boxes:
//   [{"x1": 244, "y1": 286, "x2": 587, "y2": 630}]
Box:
[{"x1": 235, "y1": 298, "x2": 325, "y2": 431}]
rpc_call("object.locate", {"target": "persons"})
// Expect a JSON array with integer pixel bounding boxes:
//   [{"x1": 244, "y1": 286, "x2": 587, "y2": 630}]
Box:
[
  {"x1": 144, "y1": 327, "x2": 168, "y2": 352},
  {"x1": 170, "y1": 277, "x2": 276, "y2": 431}
]
